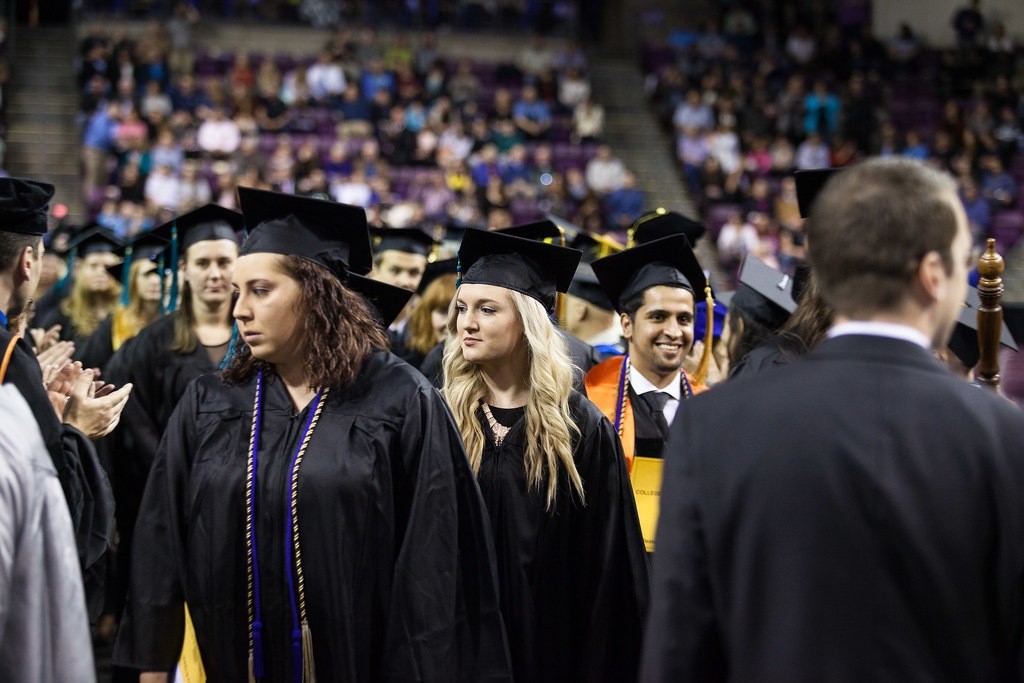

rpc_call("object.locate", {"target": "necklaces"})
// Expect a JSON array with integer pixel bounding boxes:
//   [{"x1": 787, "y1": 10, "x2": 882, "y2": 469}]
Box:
[
  {"x1": 614, "y1": 355, "x2": 693, "y2": 436},
  {"x1": 481, "y1": 402, "x2": 511, "y2": 447}
]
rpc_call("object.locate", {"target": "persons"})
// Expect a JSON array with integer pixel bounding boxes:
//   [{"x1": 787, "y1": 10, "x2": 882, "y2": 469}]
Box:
[{"x1": 1, "y1": 0, "x2": 1022, "y2": 683}]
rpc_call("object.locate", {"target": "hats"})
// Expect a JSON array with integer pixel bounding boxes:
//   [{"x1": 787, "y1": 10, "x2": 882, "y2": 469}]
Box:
[
  {"x1": 570, "y1": 261, "x2": 614, "y2": 312},
  {"x1": 791, "y1": 264, "x2": 812, "y2": 305},
  {"x1": 793, "y1": 167, "x2": 845, "y2": 219},
  {"x1": 371, "y1": 227, "x2": 444, "y2": 256},
  {"x1": 590, "y1": 233, "x2": 716, "y2": 386},
  {"x1": 955, "y1": 284, "x2": 1020, "y2": 353},
  {"x1": 456, "y1": 227, "x2": 583, "y2": 315},
  {"x1": 730, "y1": 255, "x2": 798, "y2": 332},
  {"x1": 345, "y1": 270, "x2": 415, "y2": 330},
  {"x1": 150, "y1": 203, "x2": 244, "y2": 257},
  {"x1": 492, "y1": 219, "x2": 562, "y2": 243},
  {"x1": 0, "y1": 177, "x2": 55, "y2": 235},
  {"x1": 237, "y1": 186, "x2": 373, "y2": 288},
  {"x1": 626, "y1": 206, "x2": 705, "y2": 249},
  {"x1": 415, "y1": 257, "x2": 457, "y2": 297},
  {"x1": 59, "y1": 232, "x2": 122, "y2": 259},
  {"x1": 568, "y1": 233, "x2": 601, "y2": 251},
  {"x1": 110, "y1": 235, "x2": 172, "y2": 263}
]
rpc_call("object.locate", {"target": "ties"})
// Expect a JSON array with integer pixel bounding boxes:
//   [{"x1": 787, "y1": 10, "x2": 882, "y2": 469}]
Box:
[{"x1": 640, "y1": 391, "x2": 670, "y2": 442}]
